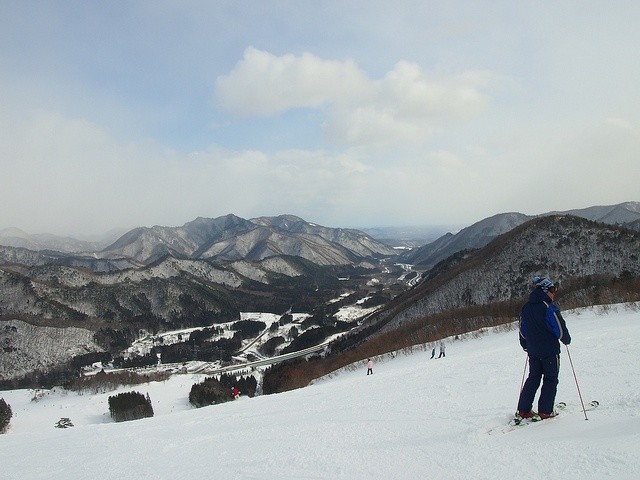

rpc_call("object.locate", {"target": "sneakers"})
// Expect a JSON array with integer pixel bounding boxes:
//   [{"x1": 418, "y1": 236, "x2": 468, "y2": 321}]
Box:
[
  {"x1": 519, "y1": 410, "x2": 536, "y2": 419},
  {"x1": 538, "y1": 411, "x2": 556, "y2": 420}
]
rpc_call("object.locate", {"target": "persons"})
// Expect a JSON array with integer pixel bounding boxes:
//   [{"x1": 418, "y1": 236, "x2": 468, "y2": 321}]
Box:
[
  {"x1": 366, "y1": 359, "x2": 373, "y2": 375},
  {"x1": 430, "y1": 347, "x2": 437, "y2": 360},
  {"x1": 517, "y1": 276, "x2": 572, "y2": 418},
  {"x1": 438, "y1": 342, "x2": 446, "y2": 358},
  {"x1": 231, "y1": 386, "x2": 240, "y2": 399}
]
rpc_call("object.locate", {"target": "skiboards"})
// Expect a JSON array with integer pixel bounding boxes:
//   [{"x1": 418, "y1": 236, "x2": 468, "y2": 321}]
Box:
[{"x1": 485, "y1": 401, "x2": 599, "y2": 436}]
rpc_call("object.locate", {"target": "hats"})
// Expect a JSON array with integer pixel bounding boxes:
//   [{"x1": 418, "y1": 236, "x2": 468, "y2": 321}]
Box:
[{"x1": 535, "y1": 277, "x2": 554, "y2": 292}]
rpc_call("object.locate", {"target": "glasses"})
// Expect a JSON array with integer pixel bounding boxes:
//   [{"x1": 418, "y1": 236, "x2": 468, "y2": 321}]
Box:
[{"x1": 546, "y1": 286, "x2": 556, "y2": 293}]
